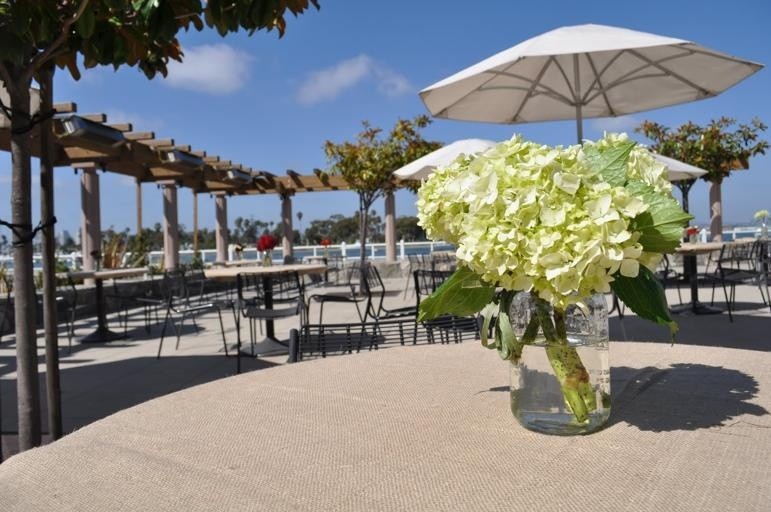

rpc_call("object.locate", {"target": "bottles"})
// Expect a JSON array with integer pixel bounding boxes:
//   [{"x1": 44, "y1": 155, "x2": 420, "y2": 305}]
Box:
[
  {"x1": 263, "y1": 249, "x2": 272, "y2": 267},
  {"x1": 324, "y1": 245, "x2": 328, "y2": 257},
  {"x1": 761, "y1": 221, "x2": 768, "y2": 240}
]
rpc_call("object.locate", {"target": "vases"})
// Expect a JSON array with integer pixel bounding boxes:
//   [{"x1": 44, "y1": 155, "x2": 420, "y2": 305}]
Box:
[
  {"x1": 323, "y1": 246, "x2": 328, "y2": 265},
  {"x1": 263, "y1": 252, "x2": 272, "y2": 266}
]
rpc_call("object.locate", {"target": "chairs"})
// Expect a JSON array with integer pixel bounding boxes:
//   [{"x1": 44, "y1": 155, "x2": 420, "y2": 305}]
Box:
[
  {"x1": 615, "y1": 235, "x2": 770, "y2": 323},
  {"x1": 0, "y1": 255, "x2": 481, "y2": 374}
]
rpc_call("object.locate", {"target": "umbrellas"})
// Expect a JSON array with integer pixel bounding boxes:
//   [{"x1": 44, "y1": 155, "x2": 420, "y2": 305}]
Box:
[
  {"x1": 387, "y1": 134, "x2": 500, "y2": 181},
  {"x1": 418, "y1": 25, "x2": 763, "y2": 170},
  {"x1": 643, "y1": 146, "x2": 710, "y2": 186}
]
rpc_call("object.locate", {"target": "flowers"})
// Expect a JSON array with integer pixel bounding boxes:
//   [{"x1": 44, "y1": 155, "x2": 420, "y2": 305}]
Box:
[
  {"x1": 414, "y1": 126, "x2": 695, "y2": 419},
  {"x1": 258, "y1": 234, "x2": 277, "y2": 251},
  {"x1": 319, "y1": 237, "x2": 332, "y2": 246}
]
